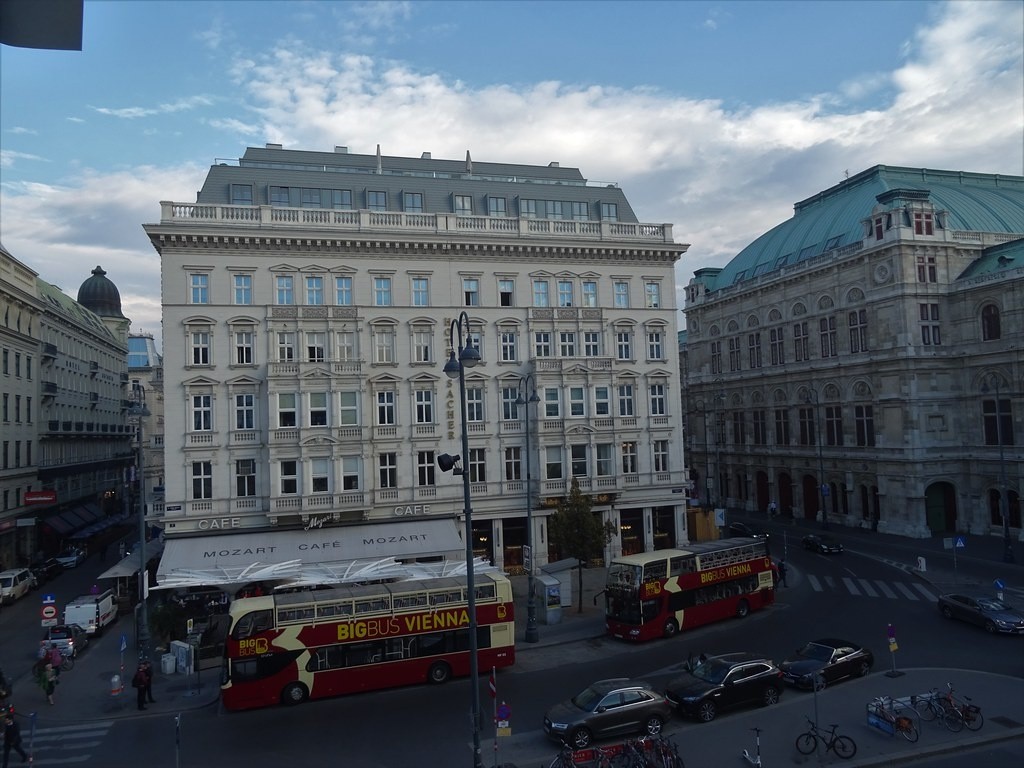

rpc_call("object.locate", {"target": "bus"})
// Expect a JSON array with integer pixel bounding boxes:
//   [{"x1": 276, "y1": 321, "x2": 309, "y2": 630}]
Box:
[
  {"x1": 219, "y1": 569, "x2": 516, "y2": 714},
  {"x1": 594, "y1": 536, "x2": 774, "y2": 643}
]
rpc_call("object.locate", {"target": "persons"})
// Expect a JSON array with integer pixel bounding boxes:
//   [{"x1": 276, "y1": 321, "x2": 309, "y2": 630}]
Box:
[
  {"x1": 792, "y1": 503, "x2": 801, "y2": 525},
  {"x1": 49, "y1": 643, "x2": 63, "y2": 684},
  {"x1": 772, "y1": 562, "x2": 779, "y2": 590},
  {"x1": 137, "y1": 661, "x2": 156, "y2": 710},
  {"x1": 777, "y1": 559, "x2": 789, "y2": 588},
  {"x1": 862, "y1": 511, "x2": 878, "y2": 535},
  {"x1": 815, "y1": 509, "x2": 823, "y2": 528},
  {"x1": 1, "y1": 715, "x2": 28, "y2": 768},
  {"x1": 99, "y1": 540, "x2": 107, "y2": 560},
  {"x1": 770, "y1": 501, "x2": 777, "y2": 519},
  {"x1": 40, "y1": 663, "x2": 58, "y2": 704}
]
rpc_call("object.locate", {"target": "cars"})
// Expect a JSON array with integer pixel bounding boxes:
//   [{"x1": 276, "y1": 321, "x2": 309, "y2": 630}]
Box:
[
  {"x1": 56, "y1": 546, "x2": 87, "y2": 570},
  {"x1": 939, "y1": 591, "x2": 1024, "y2": 637},
  {"x1": 725, "y1": 520, "x2": 772, "y2": 541},
  {"x1": 543, "y1": 676, "x2": 673, "y2": 750},
  {"x1": 662, "y1": 651, "x2": 786, "y2": 723},
  {"x1": 802, "y1": 533, "x2": 845, "y2": 556},
  {"x1": 775, "y1": 637, "x2": 875, "y2": 692}
]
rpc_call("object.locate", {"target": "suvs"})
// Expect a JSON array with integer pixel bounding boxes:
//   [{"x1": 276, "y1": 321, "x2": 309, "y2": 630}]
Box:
[
  {"x1": 42, "y1": 623, "x2": 89, "y2": 660},
  {"x1": 0, "y1": 567, "x2": 36, "y2": 608}
]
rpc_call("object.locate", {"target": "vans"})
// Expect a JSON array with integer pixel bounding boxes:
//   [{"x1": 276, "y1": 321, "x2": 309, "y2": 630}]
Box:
[{"x1": 63, "y1": 587, "x2": 120, "y2": 638}]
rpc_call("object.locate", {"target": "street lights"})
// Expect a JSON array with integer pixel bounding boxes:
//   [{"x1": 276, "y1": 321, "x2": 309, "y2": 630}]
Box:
[
  {"x1": 805, "y1": 389, "x2": 830, "y2": 532},
  {"x1": 127, "y1": 383, "x2": 152, "y2": 649},
  {"x1": 981, "y1": 373, "x2": 1016, "y2": 564},
  {"x1": 695, "y1": 399, "x2": 712, "y2": 512},
  {"x1": 711, "y1": 378, "x2": 729, "y2": 509},
  {"x1": 443, "y1": 311, "x2": 483, "y2": 768},
  {"x1": 514, "y1": 375, "x2": 541, "y2": 644}
]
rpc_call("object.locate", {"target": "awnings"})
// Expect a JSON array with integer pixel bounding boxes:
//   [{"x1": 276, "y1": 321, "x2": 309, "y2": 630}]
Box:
[
  {"x1": 43, "y1": 502, "x2": 108, "y2": 539},
  {"x1": 155, "y1": 519, "x2": 466, "y2": 590}
]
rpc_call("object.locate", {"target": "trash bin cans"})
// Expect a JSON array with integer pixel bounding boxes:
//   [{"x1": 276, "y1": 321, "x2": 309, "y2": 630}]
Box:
[{"x1": 161, "y1": 656, "x2": 176, "y2": 674}]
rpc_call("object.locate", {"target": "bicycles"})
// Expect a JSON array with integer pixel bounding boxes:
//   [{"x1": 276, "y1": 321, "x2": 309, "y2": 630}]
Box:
[
  {"x1": 914, "y1": 688, "x2": 964, "y2": 733},
  {"x1": 32, "y1": 646, "x2": 76, "y2": 677},
  {"x1": 872, "y1": 695, "x2": 919, "y2": 743},
  {"x1": 931, "y1": 682, "x2": 984, "y2": 731},
  {"x1": 796, "y1": 713, "x2": 857, "y2": 759},
  {"x1": 549, "y1": 729, "x2": 686, "y2": 768}
]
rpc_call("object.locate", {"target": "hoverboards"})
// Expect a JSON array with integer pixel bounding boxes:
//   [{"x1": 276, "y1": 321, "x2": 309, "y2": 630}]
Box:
[{"x1": 741, "y1": 726, "x2": 764, "y2": 768}]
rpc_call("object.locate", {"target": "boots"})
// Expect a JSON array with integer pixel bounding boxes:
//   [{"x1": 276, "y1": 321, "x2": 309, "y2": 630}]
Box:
[
  {"x1": 138, "y1": 705, "x2": 140, "y2": 710},
  {"x1": 148, "y1": 696, "x2": 156, "y2": 702},
  {"x1": 140, "y1": 704, "x2": 147, "y2": 710},
  {"x1": 48, "y1": 696, "x2": 54, "y2": 704}
]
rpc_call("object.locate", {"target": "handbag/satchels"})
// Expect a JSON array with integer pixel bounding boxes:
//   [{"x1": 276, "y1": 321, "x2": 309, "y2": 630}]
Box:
[{"x1": 132, "y1": 673, "x2": 143, "y2": 687}]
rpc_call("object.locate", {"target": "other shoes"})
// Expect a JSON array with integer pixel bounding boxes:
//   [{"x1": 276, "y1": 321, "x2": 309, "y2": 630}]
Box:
[{"x1": 21, "y1": 754, "x2": 28, "y2": 763}]
